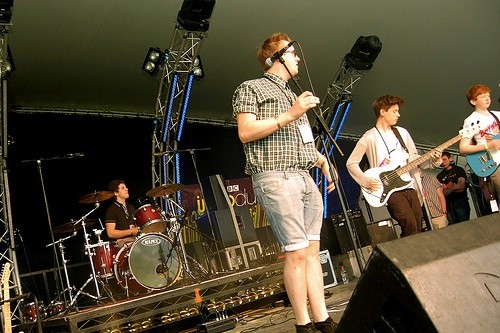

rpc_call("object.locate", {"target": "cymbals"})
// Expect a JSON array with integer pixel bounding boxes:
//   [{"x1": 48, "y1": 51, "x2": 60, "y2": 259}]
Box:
[
  {"x1": 52, "y1": 219, "x2": 97, "y2": 233},
  {"x1": 78, "y1": 191, "x2": 115, "y2": 203},
  {"x1": 145, "y1": 183, "x2": 180, "y2": 198}
]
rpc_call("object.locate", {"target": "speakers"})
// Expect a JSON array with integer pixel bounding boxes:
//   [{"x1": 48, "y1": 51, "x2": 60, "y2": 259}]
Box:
[
  {"x1": 195, "y1": 204, "x2": 257, "y2": 254},
  {"x1": 333, "y1": 212, "x2": 500, "y2": 333},
  {"x1": 344, "y1": 35, "x2": 383, "y2": 71},
  {"x1": 177, "y1": 0, "x2": 215, "y2": 31},
  {"x1": 325, "y1": 208, "x2": 372, "y2": 254}
]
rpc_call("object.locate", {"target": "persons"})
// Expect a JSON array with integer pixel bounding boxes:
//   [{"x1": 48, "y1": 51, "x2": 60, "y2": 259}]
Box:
[
  {"x1": 418, "y1": 167, "x2": 447, "y2": 229},
  {"x1": 436, "y1": 152, "x2": 471, "y2": 225},
  {"x1": 232, "y1": 33, "x2": 338, "y2": 333},
  {"x1": 104, "y1": 179, "x2": 139, "y2": 239},
  {"x1": 459, "y1": 84, "x2": 500, "y2": 211},
  {"x1": 345, "y1": 95, "x2": 443, "y2": 238}
]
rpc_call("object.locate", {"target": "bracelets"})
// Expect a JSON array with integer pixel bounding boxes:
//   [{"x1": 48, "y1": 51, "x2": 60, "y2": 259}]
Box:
[
  {"x1": 275, "y1": 115, "x2": 282, "y2": 129},
  {"x1": 443, "y1": 210, "x2": 447, "y2": 214},
  {"x1": 321, "y1": 161, "x2": 326, "y2": 167},
  {"x1": 484, "y1": 143, "x2": 489, "y2": 150}
]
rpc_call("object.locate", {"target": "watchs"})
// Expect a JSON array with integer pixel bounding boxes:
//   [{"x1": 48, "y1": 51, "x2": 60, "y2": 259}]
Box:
[{"x1": 131, "y1": 229, "x2": 133, "y2": 235}]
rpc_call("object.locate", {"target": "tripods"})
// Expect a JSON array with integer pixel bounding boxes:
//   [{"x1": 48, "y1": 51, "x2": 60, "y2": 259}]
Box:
[{"x1": 47, "y1": 220, "x2": 117, "y2": 317}]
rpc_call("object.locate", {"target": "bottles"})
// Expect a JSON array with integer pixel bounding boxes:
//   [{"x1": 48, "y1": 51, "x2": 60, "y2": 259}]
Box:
[
  {"x1": 340, "y1": 265, "x2": 349, "y2": 284},
  {"x1": 489, "y1": 194, "x2": 499, "y2": 213}
]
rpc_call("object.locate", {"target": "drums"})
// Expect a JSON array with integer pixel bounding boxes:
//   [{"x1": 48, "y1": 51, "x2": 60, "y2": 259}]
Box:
[
  {"x1": 47, "y1": 299, "x2": 70, "y2": 316},
  {"x1": 109, "y1": 241, "x2": 120, "y2": 268},
  {"x1": 133, "y1": 203, "x2": 167, "y2": 234},
  {"x1": 23, "y1": 300, "x2": 45, "y2": 323},
  {"x1": 113, "y1": 233, "x2": 181, "y2": 294},
  {"x1": 89, "y1": 241, "x2": 114, "y2": 278}
]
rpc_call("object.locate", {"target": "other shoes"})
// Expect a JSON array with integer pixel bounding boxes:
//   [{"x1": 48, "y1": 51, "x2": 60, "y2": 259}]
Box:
[
  {"x1": 294, "y1": 321, "x2": 322, "y2": 333},
  {"x1": 313, "y1": 316, "x2": 339, "y2": 333}
]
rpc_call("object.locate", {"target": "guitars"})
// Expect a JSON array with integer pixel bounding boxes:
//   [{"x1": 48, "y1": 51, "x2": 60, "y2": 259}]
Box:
[
  {"x1": 360, "y1": 128, "x2": 465, "y2": 208},
  {"x1": 465, "y1": 132, "x2": 500, "y2": 178},
  {"x1": 0, "y1": 262, "x2": 13, "y2": 333}
]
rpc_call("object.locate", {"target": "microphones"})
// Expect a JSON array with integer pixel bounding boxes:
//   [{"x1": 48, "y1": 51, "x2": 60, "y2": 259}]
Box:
[
  {"x1": 64, "y1": 152, "x2": 86, "y2": 158},
  {"x1": 153, "y1": 152, "x2": 168, "y2": 157},
  {"x1": 264, "y1": 40, "x2": 295, "y2": 68}
]
